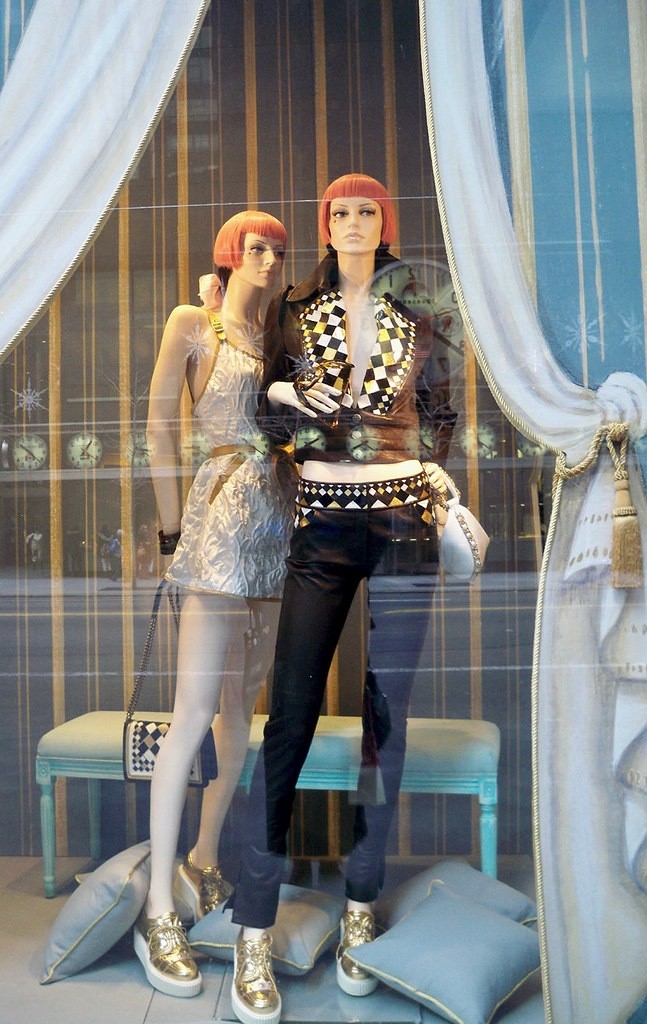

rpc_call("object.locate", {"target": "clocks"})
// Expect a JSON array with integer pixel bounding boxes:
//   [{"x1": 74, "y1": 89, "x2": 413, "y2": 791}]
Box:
[
  {"x1": 233, "y1": 425, "x2": 270, "y2": 465},
  {"x1": 12, "y1": 433, "x2": 47, "y2": 470},
  {"x1": 458, "y1": 421, "x2": 497, "y2": 459},
  {"x1": 355, "y1": 258, "x2": 468, "y2": 390},
  {"x1": 121, "y1": 428, "x2": 159, "y2": 468},
  {"x1": 66, "y1": 431, "x2": 103, "y2": 469},
  {"x1": 515, "y1": 425, "x2": 550, "y2": 457},
  {"x1": 345, "y1": 424, "x2": 386, "y2": 463},
  {"x1": 175, "y1": 428, "x2": 214, "y2": 467},
  {"x1": 400, "y1": 422, "x2": 441, "y2": 460},
  {"x1": 291, "y1": 425, "x2": 327, "y2": 463}
]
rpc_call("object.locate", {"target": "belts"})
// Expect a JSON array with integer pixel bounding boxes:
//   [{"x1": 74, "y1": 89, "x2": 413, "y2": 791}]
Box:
[{"x1": 208, "y1": 443, "x2": 296, "y2": 506}]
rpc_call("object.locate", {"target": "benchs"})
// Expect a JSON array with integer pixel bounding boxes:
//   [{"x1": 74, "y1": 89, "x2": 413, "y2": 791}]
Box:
[{"x1": 35, "y1": 710, "x2": 500, "y2": 899}]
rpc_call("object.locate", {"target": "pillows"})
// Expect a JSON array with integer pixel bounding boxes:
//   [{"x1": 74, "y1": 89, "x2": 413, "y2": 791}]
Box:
[
  {"x1": 346, "y1": 878, "x2": 540, "y2": 1024},
  {"x1": 373, "y1": 854, "x2": 540, "y2": 934},
  {"x1": 74, "y1": 851, "x2": 194, "y2": 925},
  {"x1": 187, "y1": 884, "x2": 345, "y2": 976},
  {"x1": 39, "y1": 839, "x2": 154, "y2": 985}
]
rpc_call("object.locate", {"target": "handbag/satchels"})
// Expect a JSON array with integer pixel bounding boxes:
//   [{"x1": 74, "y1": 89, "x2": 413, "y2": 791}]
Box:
[
  {"x1": 424, "y1": 462, "x2": 491, "y2": 583},
  {"x1": 122, "y1": 718, "x2": 217, "y2": 788}
]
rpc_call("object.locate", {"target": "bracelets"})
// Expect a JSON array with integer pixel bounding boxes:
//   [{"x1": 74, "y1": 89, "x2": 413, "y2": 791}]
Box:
[{"x1": 159, "y1": 530, "x2": 181, "y2": 555}]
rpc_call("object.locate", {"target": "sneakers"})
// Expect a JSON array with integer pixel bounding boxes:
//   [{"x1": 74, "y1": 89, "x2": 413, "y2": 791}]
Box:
[
  {"x1": 230, "y1": 925, "x2": 281, "y2": 1024},
  {"x1": 173, "y1": 855, "x2": 232, "y2": 920},
  {"x1": 337, "y1": 901, "x2": 378, "y2": 995},
  {"x1": 131, "y1": 908, "x2": 202, "y2": 997}
]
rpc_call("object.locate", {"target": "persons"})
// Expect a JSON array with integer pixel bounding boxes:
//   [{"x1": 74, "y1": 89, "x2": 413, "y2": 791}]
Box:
[
  {"x1": 221, "y1": 174, "x2": 458, "y2": 1023},
  {"x1": 25, "y1": 526, "x2": 165, "y2": 581},
  {"x1": 123, "y1": 211, "x2": 292, "y2": 996}
]
